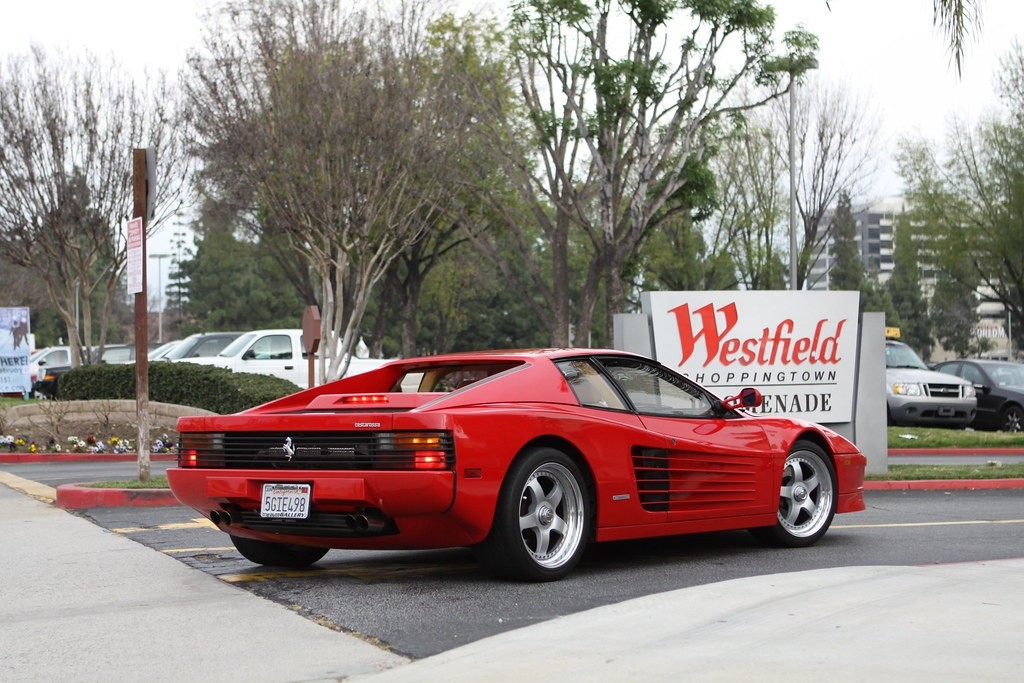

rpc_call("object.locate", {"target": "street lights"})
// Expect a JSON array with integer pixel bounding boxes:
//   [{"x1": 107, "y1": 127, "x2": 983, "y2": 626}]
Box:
[{"x1": 149, "y1": 254, "x2": 168, "y2": 343}]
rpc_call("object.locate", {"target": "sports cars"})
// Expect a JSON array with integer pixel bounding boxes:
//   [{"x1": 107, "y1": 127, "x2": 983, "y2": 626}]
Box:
[{"x1": 166, "y1": 348, "x2": 868, "y2": 583}]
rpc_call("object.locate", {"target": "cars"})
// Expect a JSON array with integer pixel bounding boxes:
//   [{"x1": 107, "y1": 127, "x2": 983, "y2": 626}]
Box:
[
  {"x1": 30, "y1": 346, "x2": 94, "y2": 379},
  {"x1": 885, "y1": 327, "x2": 976, "y2": 430},
  {"x1": 926, "y1": 359, "x2": 1024, "y2": 432},
  {"x1": 31, "y1": 342, "x2": 163, "y2": 399},
  {"x1": 156, "y1": 333, "x2": 244, "y2": 363}
]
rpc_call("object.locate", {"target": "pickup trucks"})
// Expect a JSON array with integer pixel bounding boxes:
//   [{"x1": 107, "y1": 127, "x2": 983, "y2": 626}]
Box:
[{"x1": 211, "y1": 329, "x2": 424, "y2": 393}]
